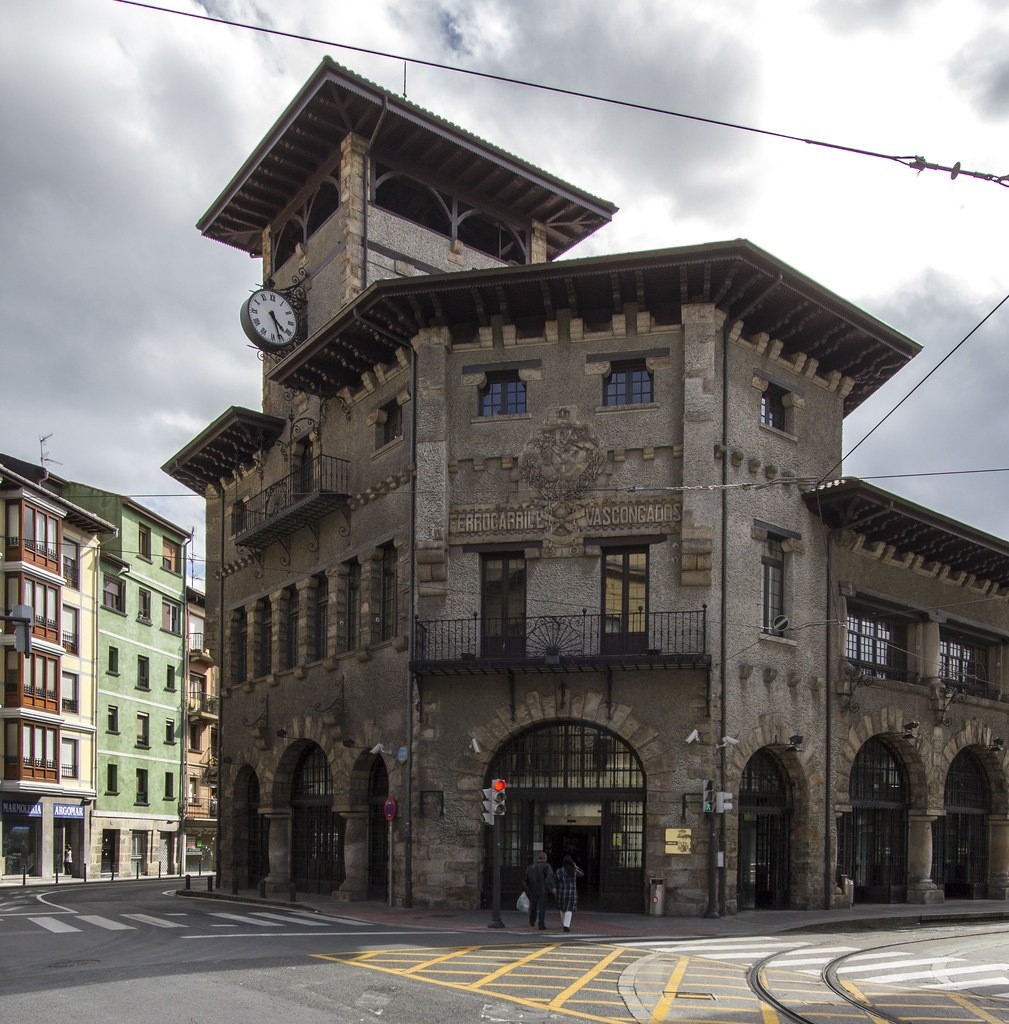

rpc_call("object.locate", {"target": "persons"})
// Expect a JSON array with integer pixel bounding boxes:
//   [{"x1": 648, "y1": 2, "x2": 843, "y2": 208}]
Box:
[
  {"x1": 553, "y1": 856, "x2": 585, "y2": 933},
  {"x1": 521, "y1": 852, "x2": 558, "y2": 931},
  {"x1": 64, "y1": 844, "x2": 73, "y2": 874}
]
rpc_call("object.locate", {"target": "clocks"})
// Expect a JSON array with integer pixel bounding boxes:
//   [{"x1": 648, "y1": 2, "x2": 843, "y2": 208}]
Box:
[{"x1": 241, "y1": 289, "x2": 298, "y2": 350}]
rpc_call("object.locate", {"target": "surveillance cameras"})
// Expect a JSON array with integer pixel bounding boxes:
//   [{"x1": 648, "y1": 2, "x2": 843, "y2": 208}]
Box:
[
  {"x1": 685, "y1": 729, "x2": 698, "y2": 745},
  {"x1": 723, "y1": 735, "x2": 741, "y2": 744},
  {"x1": 472, "y1": 738, "x2": 482, "y2": 755},
  {"x1": 369, "y1": 744, "x2": 384, "y2": 755}
]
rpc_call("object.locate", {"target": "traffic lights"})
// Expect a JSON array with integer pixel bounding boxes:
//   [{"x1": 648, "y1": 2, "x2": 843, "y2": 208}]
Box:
[
  {"x1": 483, "y1": 788, "x2": 495, "y2": 825},
  {"x1": 716, "y1": 792, "x2": 733, "y2": 813},
  {"x1": 703, "y1": 780, "x2": 715, "y2": 812},
  {"x1": 494, "y1": 780, "x2": 506, "y2": 814}
]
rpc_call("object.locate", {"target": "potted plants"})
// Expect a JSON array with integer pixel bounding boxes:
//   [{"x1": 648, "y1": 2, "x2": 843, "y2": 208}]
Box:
[{"x1": 208, "y1": 696, "x2": 218, "y2": 715}]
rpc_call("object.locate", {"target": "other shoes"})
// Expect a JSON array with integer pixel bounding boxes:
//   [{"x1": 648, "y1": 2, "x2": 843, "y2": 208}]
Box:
[
  {"x1": 529, "y1": 921, "x2": 535, "y2": 927},
  {"x1": 539, "y1": 925, "x2": 546, "y2": 930},
  {"x1": 563, "y1": 926, "x2": 571, "y2": 933}
]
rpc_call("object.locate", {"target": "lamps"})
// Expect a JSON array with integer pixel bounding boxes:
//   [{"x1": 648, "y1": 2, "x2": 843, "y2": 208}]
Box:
[
  {"x1": 990, "y1": 738, "x2": 1004, "y2": 751},
  {"x1": 343, "y1": 738, "x2": 372, "y2": 749},
  {"x1": 782, "y1": 735, "x2": 803, "y2": 745},
  {"x1": 224, "y1": 755, "x2": 245, "y2": 766},
  {"x1": 277, "y1": 729, "x2": 305, "y2": 740},
  {"x1": 902, "y1": 723, "x2": 920, "y2": 739}
]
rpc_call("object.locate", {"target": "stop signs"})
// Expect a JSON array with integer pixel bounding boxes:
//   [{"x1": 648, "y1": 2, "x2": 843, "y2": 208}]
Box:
[{"x1": 773, "y1": 615, "x2": 789, "y2": 631}]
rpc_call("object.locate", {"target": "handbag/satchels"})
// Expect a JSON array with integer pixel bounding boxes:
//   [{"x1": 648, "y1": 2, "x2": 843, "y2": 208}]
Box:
[{"x1": 516, "y1": 892, "x2": 530, "y2": 913}]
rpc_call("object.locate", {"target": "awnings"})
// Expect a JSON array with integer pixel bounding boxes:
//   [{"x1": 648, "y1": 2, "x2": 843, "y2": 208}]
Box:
[{"x1": 184, "y1": 816, "x2": 218, "y2": 834}]
